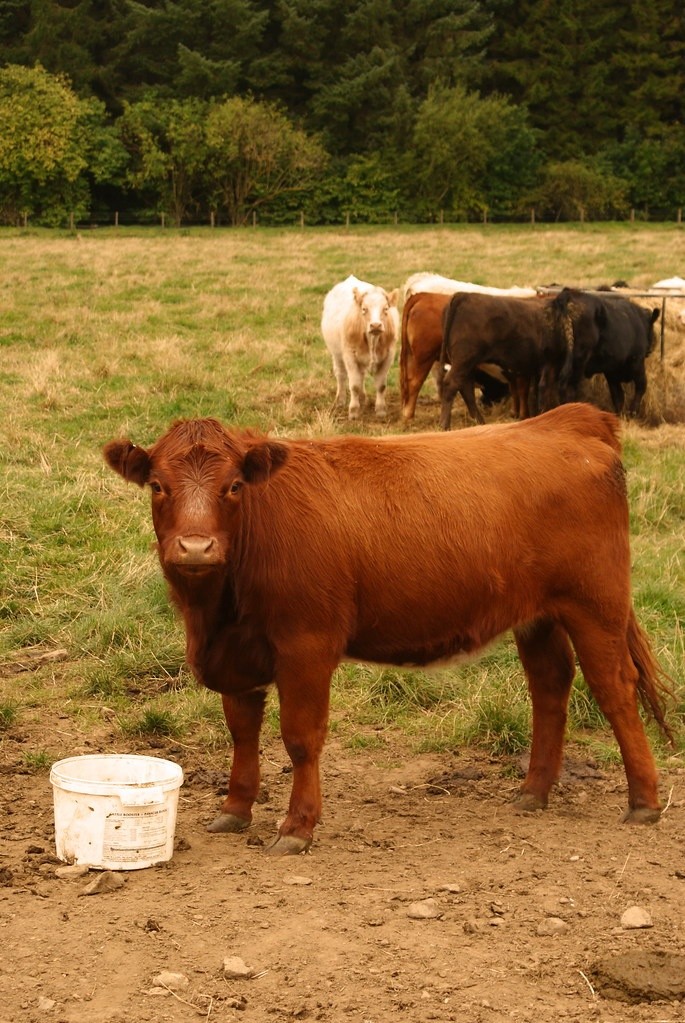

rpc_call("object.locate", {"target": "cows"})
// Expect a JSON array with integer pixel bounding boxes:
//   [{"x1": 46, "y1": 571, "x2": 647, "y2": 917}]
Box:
[
  {"x1": 102, "y1": 400, "x2": 674, "y2": 860},
  {"x1": 321, "y1": 272, "x2": 685, "y2": 432}
]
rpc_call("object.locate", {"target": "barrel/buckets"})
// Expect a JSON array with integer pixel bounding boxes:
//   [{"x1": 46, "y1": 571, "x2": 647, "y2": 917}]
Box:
[{"x1": 49, "y1": 753, "x2": 185, "y2": 871}]
